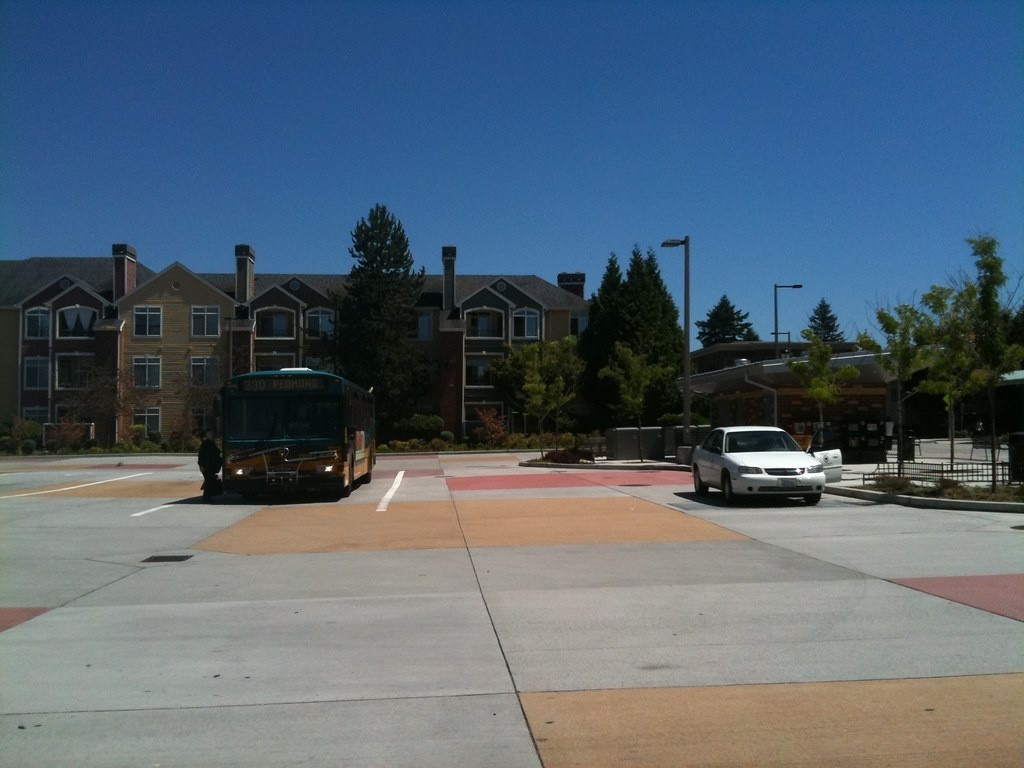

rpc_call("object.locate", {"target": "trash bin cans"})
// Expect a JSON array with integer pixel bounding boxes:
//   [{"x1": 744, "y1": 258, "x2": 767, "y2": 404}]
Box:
[
  {"x1": 606, "y1": 425, "x2": 666, "y2": 460},
  {"x1": 674, "y1": 424, "x2": 713, "y2": 459}
]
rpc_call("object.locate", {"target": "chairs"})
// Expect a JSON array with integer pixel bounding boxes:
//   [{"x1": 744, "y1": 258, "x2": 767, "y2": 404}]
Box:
[{"x1": 729, "y1": 437, "x2": 738, "y2": 451}]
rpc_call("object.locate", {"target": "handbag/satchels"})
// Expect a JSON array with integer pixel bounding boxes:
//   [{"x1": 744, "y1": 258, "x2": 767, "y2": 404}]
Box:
[{"x1": 208, "y1": 479, "x2": 224, "y2": 496}]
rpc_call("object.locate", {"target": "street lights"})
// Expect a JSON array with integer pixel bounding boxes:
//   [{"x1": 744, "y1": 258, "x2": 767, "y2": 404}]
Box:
[
  {"x1": 774, "y1": 283, "x2": 803, "y2": 359},
  {"x1": 662, "y1": 235, "x2": 691, "y2": 447}
]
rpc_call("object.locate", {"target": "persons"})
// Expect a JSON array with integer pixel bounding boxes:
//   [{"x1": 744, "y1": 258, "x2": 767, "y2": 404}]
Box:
[{"x1": 198, "y1": 428, "x2": 221, "y2": 501}]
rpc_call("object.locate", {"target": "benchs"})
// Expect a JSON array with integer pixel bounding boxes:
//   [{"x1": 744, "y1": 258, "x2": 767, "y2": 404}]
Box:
[{"x1": 969, "y1": 432, "x2": 1002, "y2": 461}]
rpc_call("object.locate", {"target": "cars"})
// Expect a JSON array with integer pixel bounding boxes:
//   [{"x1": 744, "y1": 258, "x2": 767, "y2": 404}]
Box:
[{"x1": 692, "y1": 425, "x2": 842, "y2": 505}]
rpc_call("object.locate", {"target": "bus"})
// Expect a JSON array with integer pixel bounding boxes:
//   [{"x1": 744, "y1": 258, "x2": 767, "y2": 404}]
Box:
[{"x1": 221, "y1": 368, "x2": 376, "y2": 498}]
